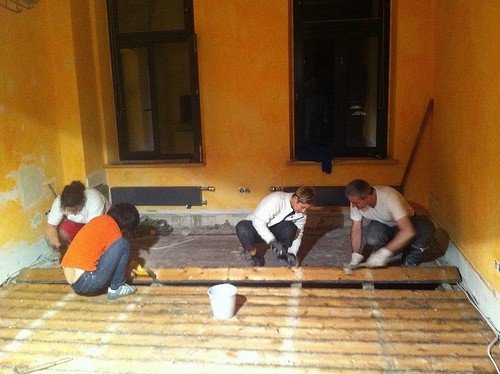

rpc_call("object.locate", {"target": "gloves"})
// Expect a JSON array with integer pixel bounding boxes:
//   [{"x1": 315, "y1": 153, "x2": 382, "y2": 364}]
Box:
[
  {"x1": 269, "y1": 241, "x2": 296, "y2": 269},
  {"x1": 346, "y1": 252, "x2": 364, "y2": 269},
  {"x1": 367, "y1": 248, "x2": 396, "y2": 268}
]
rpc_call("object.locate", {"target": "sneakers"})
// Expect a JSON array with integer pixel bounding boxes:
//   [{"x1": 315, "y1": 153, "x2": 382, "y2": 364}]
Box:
[{"x1": 107, "y1": 283, "x2": 137, "y2": 300}]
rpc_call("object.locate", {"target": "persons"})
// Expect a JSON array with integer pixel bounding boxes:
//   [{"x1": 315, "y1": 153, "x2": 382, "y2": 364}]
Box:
[
  {"x1": 44, "y1": 180, "x2": 112, "y2": 252},
  {"x1": 60, "y1": 202, "x2": 140, "y2": 299},
  {"x1": 235, "y1": 185, "x2": 316, "y2": 267},
  {"x1": 345, "y1": 179, "x2": 436, "y2": 271}
]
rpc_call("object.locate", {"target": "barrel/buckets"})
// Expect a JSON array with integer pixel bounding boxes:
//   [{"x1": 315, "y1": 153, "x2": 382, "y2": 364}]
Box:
[{"x1": 207, "y1": 284, "x2": 238, "y2": 320}]
